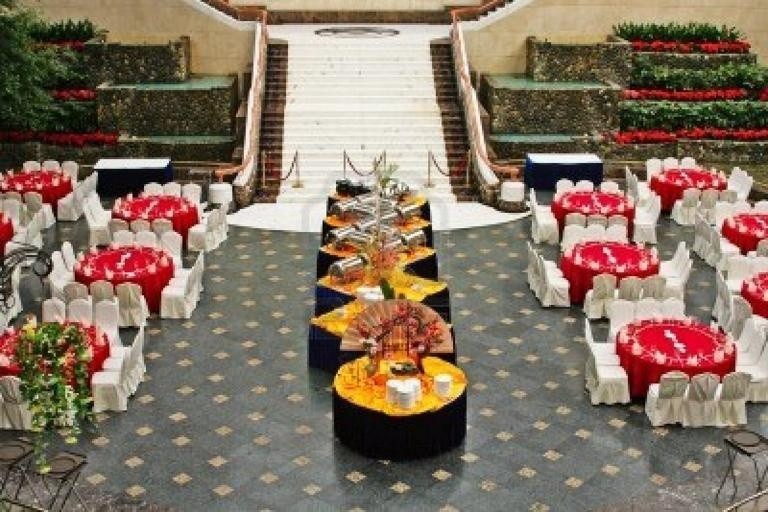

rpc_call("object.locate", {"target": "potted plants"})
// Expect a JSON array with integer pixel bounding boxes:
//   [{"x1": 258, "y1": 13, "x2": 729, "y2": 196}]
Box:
[
  {"x1": 611, "y1": 18, "x2": 768, "y2": 144},
  {"x1": 1, "y1": 18, "x2": 117, "y2": 141}
]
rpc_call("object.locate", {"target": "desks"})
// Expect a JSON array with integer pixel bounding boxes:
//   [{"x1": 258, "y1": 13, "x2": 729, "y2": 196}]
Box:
[
  {"x1": 306, "y1": 191, "x2": 466, "y2": 463},
  {"x1": 526, "y1": 151, "x2": 603, "y2": 189},
  {"x1": 93, "y1": 155, "x2": 173, "y2": 197}
]
